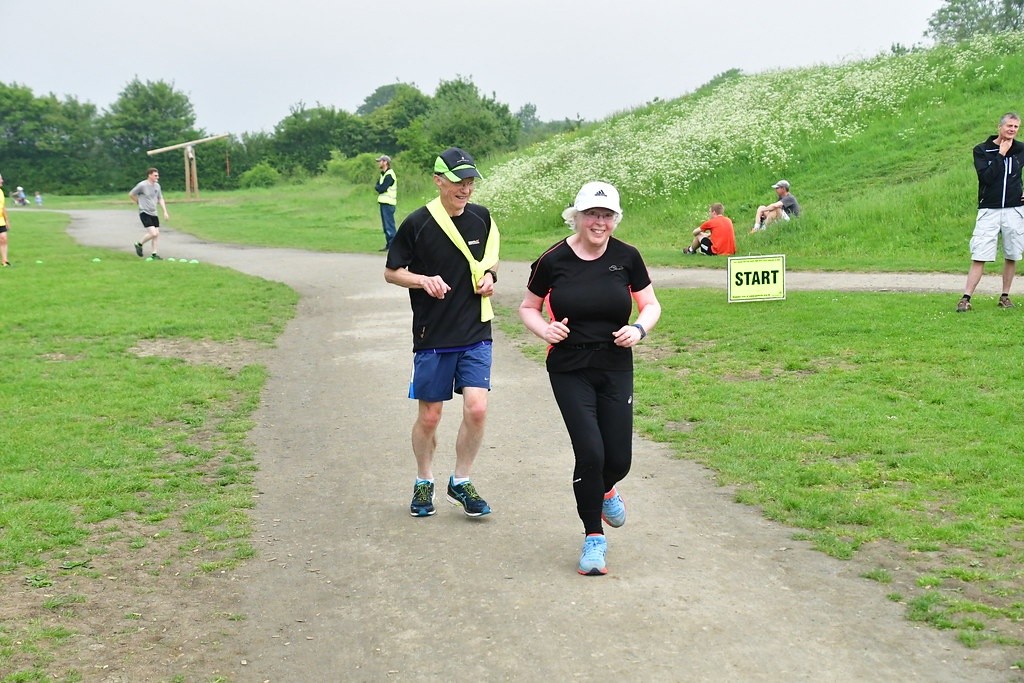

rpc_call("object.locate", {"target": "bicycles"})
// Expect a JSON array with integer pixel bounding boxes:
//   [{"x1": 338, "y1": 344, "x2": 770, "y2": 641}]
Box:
[{"x1": 9, "y1": 191, "x2": 26, "y2": 207}]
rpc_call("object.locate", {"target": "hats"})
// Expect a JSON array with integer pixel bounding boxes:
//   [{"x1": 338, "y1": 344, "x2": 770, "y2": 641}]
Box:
[
  {"x1": 17, "y1": 187, "x2": 23, "y2": 191},
  {"x1": 434, "y1": 147, "x2": 483, "y2": 183},
  {"x1": 772, "y1": 180, "x2": 789, "y2": 188},
  {"x1": 375, "y1": 155, "x2": 391, "y2": 165},
  {"x1": 574, "y1": 182, "x2": 621, "y2": 212}
]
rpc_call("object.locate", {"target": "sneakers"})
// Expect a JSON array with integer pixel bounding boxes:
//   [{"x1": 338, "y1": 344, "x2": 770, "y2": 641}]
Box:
[
  {"x1": 999, "y1": 294, "x2": 1014, "y2": 308},
  {"x1": 151, "y1": 253, "x2": 163, "y2": 261},
  {"x1": 446, "y1": 475, "x2": 491, "y2": 517},
  {"x1": 578, "y1": 534, "x2": 607, "y2": 575},
  {"x1": 134, "y1": 241, "x2": 144, "y2": 256},
  {"x1": 602, "y1": 488, "x2": 627, "y2": 528},
  {"x1": 956, "y1": 295, "x2": 973, "y2": 312},
  {"x1": 410, "y1": 477, "x2": 436, "y2": 517}
]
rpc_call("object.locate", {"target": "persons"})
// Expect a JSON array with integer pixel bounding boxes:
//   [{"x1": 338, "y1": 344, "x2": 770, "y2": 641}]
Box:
[
  {"x1": 683, "y1": 203, "x2": 736, "y2": 256},
  {"x1": 11, "y1": 186, "x2": 26, "y2": 206},
  {"x1": 518, "y1": 181, "x2": 662, "y2": 572},
  {"x1": 128, "y1": 167, "x2": 170, "y2": 260},
  {"x1": 383, "y1": 147, "x2": 502, "y2": 520},
  {"x1": 34, "y1": 191, "x2": 43, "y2": 209},
  {"x1": 0, "y1": 173, "x2": 11, "y2": 267},
  {"x1": 749, "y1": 179, "x2": 800, "y2": 234},
  {"x1": 375, "y1": 154, "x2": 397, "y2": 252},
  {"x1": 955, "y1": 113, "x2": 1024, "y2": 312}
]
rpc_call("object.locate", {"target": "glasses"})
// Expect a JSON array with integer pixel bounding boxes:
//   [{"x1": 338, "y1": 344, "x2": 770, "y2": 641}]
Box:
[
  {"x1": 576, "y1": 211, "x2": 615, "y2": 220},
  {"x1": 151, "y1": 174, "x2": 159, "y2": 178}
]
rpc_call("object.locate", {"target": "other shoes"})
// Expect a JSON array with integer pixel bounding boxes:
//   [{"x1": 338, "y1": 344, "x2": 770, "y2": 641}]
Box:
[
  {"x1": 1, "y1": 262, "x2": 11, "y2": 266},
  {"x1": 750, "y1": 227, "x2": 756, "y2": 233},
  {"x1": 698, "y1": 246, "x2": 707, "y2": 253},
  {"x1": 378, "y1": 247, "x2": 388, "y2": 251},
  {"x1": 683, "y1": 246, "x2": 696, "y2": 254}
]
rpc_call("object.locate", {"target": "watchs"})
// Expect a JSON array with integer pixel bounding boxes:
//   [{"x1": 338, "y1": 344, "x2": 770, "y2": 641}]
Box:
[
  {"x1": 631, "y1": 324, "x2": 647, "y2": 341},
  {"x1": 484, "y1": 269, "x2": 497, "y2": 284}
]
